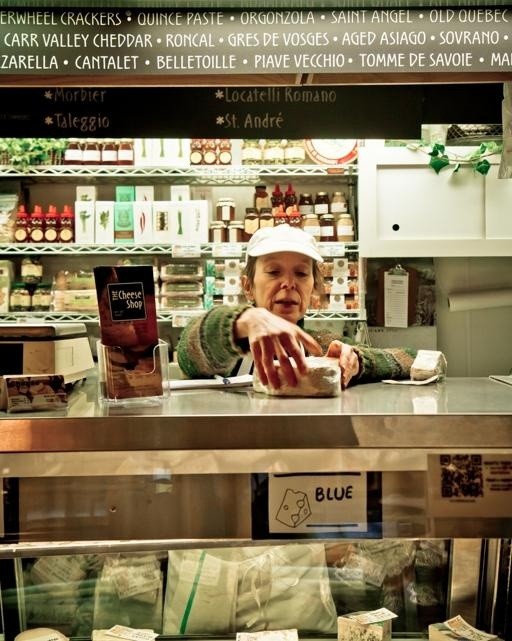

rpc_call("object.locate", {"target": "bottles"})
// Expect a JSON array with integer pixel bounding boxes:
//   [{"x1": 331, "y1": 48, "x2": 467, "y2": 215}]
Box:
[
  {"x1": 13, "y1": 204, "x2": 74, "y2": 244},
  {"x1": 188, "y1": 139, "x2": 308, "y2": 166},
  {"x1": 10, "y1": 255, "x2": 51, "y2": 310},
  {"x1": 64, "y1": 142, "x2": 135, "y2": 164},
  {"x1": 208, "y1": 184, "x2": 356, "y2": 244}
]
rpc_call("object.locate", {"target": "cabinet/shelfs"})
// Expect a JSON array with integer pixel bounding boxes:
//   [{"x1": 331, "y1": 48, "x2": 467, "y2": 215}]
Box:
[
  {"x1": 0, "y1": 162, "x2": 368, "y2": 325},
  {"x1": 357, "y1": 145, "x2": 512, "y2": 259}
]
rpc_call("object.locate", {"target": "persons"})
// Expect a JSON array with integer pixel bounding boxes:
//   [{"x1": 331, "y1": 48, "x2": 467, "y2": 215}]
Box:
[{"x1": 176, "y1": 223, "x2": 417, "y2": 392}]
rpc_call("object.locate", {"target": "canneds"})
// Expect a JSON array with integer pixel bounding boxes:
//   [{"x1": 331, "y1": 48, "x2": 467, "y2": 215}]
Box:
[
  {"x1": 210, "y1": 183, "x2": 355, "y2": 242},
  {"x1": 63, "y1": 138, "x2": 133, "y2": 165},
  {"x1": 190, "y1": 138, "x2": 306, "y2": 165},
  {"x1": 9, "y1": 254, "x2": 53, "y2": 318}
]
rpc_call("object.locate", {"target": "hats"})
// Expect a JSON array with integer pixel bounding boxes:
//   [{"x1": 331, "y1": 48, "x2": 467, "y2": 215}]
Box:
[{"x1": 246, "y1": 224, "x2": 324, "y2": 265}]
